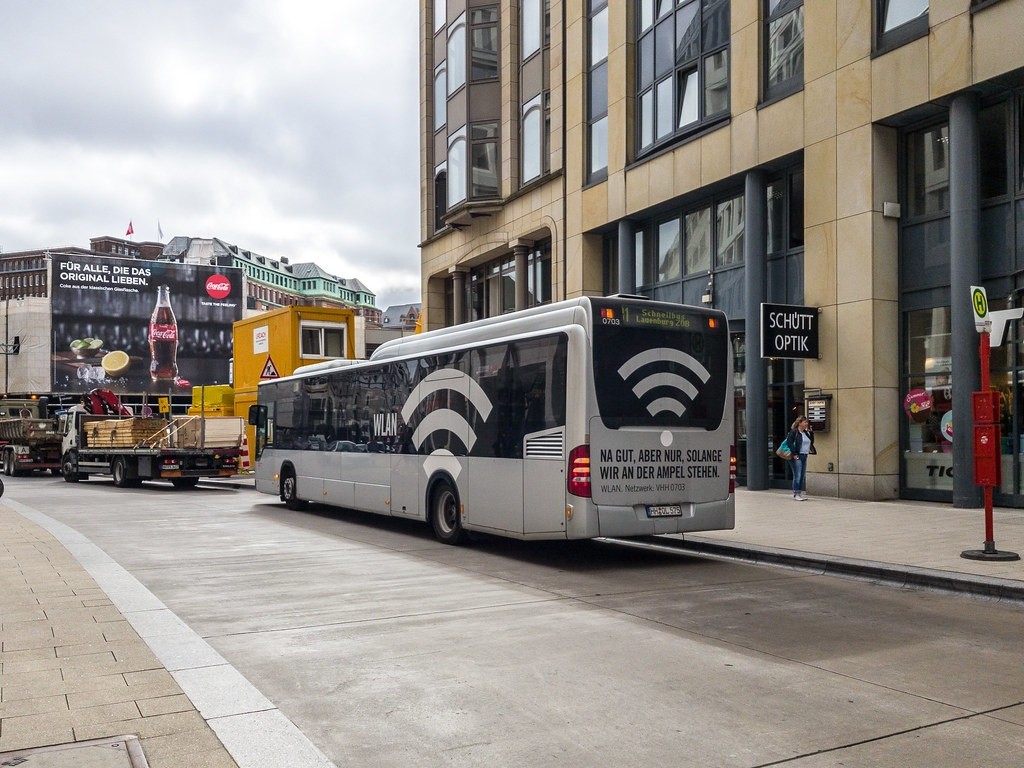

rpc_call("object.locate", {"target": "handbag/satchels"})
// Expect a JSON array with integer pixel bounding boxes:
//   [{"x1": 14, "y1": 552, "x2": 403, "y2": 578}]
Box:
[{"x1": 776, "y1": 439, "x2": 795, "y2": 460}]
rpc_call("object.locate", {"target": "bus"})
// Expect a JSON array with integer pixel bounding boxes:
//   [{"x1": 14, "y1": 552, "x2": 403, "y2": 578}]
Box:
[{"x1": 247, "y1": 293, "x2": 734, "y2": 546}]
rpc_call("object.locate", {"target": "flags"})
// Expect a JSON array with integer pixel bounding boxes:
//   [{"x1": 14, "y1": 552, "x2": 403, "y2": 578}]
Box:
[
  {"x1": 126, "y1": 221, "x2": 133, "y2": 236},
  {"x1": 158, "y1": 221, "x2": 163, "y2": 239}
]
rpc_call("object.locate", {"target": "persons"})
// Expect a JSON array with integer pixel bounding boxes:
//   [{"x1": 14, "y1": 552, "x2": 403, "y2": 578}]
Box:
[
  {"x1": 786, "y1": 416, "x2": 817, "y2": 501},
  {"x1": 931, "y1": 375, "x2": 953, "y2": 454}
]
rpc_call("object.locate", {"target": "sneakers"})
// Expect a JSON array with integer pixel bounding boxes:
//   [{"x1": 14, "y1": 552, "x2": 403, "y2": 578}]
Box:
[{"x1": 793, "y1": 494, "x2": 808, "y2": 500}]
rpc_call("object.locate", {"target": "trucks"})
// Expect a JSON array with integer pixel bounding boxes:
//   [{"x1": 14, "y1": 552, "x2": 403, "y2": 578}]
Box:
[
  {"x1": 56, "y1": 404, "x2": 244, "y2": 489},
  {"x1": 0, "y1": 399, "x2": 64, "y2": 477}
]
